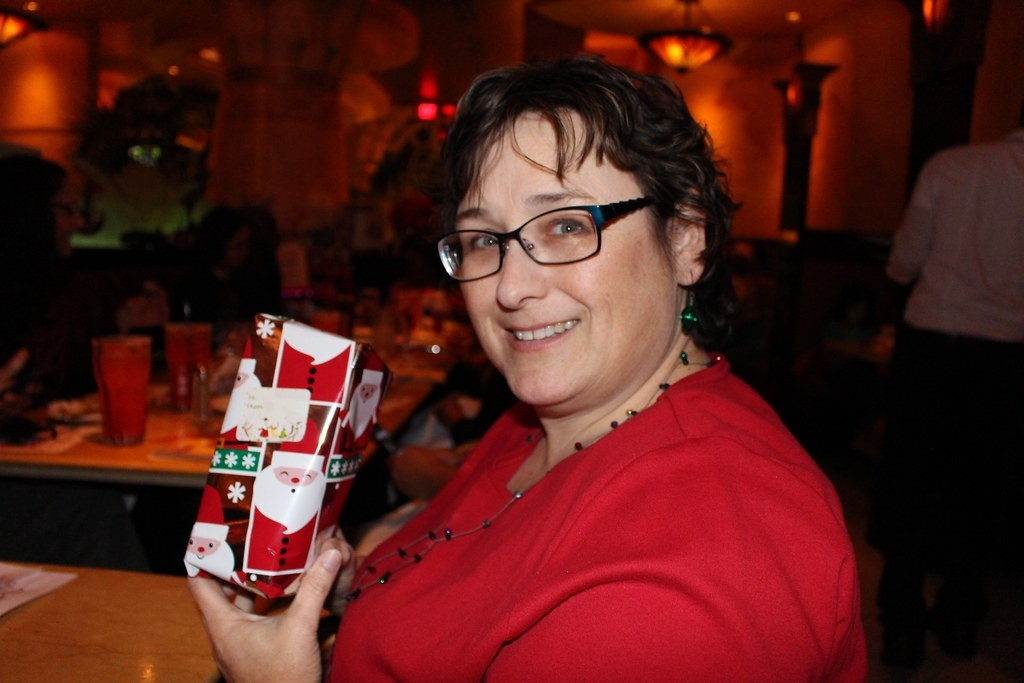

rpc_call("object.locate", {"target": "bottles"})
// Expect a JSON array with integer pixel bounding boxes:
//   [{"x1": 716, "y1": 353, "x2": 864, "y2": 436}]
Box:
[
  {"x1": 189, "y1": 363, "x2": 215, "y2": 429},
  {"x1": 292, "y1": 284, "x2": 456, "y2": 373}
]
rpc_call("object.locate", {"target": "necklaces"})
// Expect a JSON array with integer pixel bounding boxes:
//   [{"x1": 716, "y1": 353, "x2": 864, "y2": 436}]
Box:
[{"x1": 348, "y1": 331, "x2": 718, "y2": 600}]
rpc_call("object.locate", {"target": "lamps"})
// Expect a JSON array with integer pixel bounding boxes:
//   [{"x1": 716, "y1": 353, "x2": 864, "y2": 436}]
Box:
[{"x1": 641, "y1": 1, "x2": 733, "y2": 76}]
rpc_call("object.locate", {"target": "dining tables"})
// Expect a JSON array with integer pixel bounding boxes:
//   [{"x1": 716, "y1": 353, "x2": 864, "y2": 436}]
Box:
[
  {"x1": 1, "y1": 307, "x2": 459, "y2": 502},
  {"x1": 0, "y1": 553, "x2": 248, "y2": 682}
]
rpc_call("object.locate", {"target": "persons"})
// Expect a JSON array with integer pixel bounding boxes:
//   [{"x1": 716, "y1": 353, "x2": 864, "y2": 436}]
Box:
[
  {"x1": 0, "y1": 346, "x2": 53, "y2": 431},
  {"x1": 187, "y1": 51, "x2": 868, "y2": 683},
  {"x1": 0, "y1": 146, "x2": 170, "y2": 402},
  {"x1": 870, "y1": 106, "x2": 1023, "y2": 683},
  {"x1": 817, "y1": 279, "x2": 886, "y2": 473},
  {"x1": 177, "y1": 203, "x2": 285, "y2": 353},
  {"x1": 338, "y1": 437, "x2": 482, "y2": 533},
  {"x1": 418, "y1": 367, "x2": 519, "y2": 445}
]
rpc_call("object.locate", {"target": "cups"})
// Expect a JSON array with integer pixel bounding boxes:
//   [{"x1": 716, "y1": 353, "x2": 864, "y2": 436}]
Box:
[
  {"x1": 165, "y1": 319, "x2": 214, "y2": 413},
  {"x1": 89, "y1": 334, "x2": 150, "y2": 448}
]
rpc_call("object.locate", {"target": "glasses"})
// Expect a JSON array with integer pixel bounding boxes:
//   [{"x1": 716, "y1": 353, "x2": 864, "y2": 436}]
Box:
[{"x1": 437, "y1": 194, "x2": 670, "y2": 282}]
